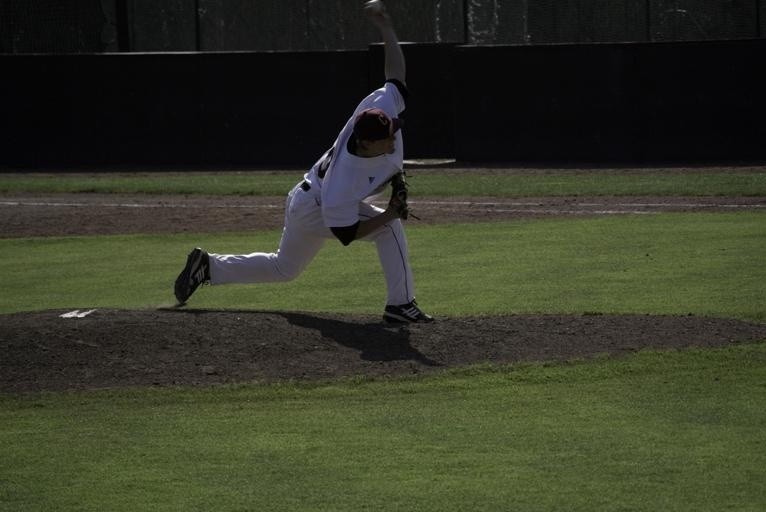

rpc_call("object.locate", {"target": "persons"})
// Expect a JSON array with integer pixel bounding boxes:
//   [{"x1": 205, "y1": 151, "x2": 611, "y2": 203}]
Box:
[{"x1": 173, "y1": 0, "x2": 435, "y2": 323}]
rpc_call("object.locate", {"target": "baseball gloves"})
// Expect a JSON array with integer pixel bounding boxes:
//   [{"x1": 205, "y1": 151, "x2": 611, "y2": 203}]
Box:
[{"x1": 389, "y1": 171, "x2": 409, "y2": 220}]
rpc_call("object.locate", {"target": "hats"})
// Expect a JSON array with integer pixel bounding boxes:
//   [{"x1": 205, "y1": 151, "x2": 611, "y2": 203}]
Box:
[{"x1": 353, "y1": 107, "x2": 404, "y2": 141}]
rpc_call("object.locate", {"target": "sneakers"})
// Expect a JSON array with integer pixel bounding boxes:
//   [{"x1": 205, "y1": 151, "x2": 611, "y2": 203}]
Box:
[
  {"x1": 383, "y1": 299, "x2": 432, "y2": 323},
  {"x1": 175, "y1": 247, "x2": 209, "y2": 303}
]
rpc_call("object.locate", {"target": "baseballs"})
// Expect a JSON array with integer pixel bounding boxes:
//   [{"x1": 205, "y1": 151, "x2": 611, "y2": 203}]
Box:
[{"x1": 371, "y1": 1, "x2": 381, "y2": 14}]
